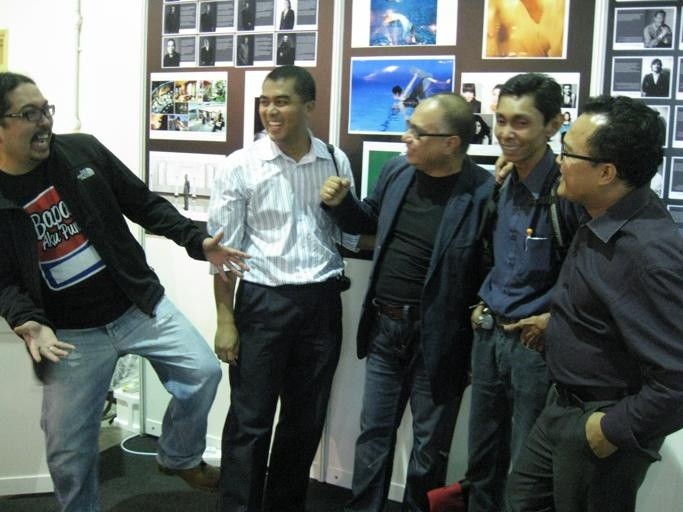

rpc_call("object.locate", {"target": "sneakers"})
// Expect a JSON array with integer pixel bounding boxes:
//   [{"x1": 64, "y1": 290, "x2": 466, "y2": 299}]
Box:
[{"x1": 158, "y1": 460, "x2": 221, "y2": 497}]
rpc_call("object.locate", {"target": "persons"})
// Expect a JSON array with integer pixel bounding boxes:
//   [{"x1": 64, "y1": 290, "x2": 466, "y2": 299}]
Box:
[
  {"x1": 561, "y1": 84, "x2": 577, "y2": 108},
  {"x1": 560, "y1": 111, "x2": 572, "y2": 135},
  {"x1": 0, "y1": 70, "x2": 252, "y2": 512},
  {"x1": 204, "y1": 62, "x2": 360, "y2": 512},
  {"x1": 643, "y1": 9, "x2": 673, "y2": 48},
  {"x1": 642, "y1": 58, "x2": 669, "y2": 97},
  {"x1": 392, "y1": 74, "x2": 417, "y2": 103},
  {"x1": 462, "y1": 83, "x2": 481, "y2": 114},
  {"x1": 494, "y1": 94, "x2": 683, "y2": 512},
  {"x1": 160, "y1": 0, "x2": 297, "y2": 67},
  {"x1": 470, "y1": 114, "x2": 492, "y2": 145},
  {"x1": 466, "y1": 72, "x2": 592, "y2": 511},
  {"x1": 488, "y1": 84, "x2": 503, "y2": 112},
  {"x1": 316, "y1": 93, "x2": 492, "y2": 512},
  {"x1": 182, "y1": 173, "x2": 190, "y2": 211}
]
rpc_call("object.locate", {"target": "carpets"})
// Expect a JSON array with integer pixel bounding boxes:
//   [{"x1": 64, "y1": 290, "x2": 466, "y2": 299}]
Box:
[{"x1": 0, "y1": 433, "x2": 409, "y2": 509}]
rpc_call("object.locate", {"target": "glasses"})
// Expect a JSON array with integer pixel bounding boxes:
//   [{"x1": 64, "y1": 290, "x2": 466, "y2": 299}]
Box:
[
  {"x1": 560, "y1": 144, "x2": 597, "y2": 162},
  {"x1": 406, "y1": 120, "x2": 452, "y2": 139},
  {"x1": 3, "y1": 105, "x2": 54, "y2": 121}
]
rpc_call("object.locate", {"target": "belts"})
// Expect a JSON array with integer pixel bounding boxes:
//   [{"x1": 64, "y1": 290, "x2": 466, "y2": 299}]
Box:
[
  {"x1": 551, "y1": 383, "x2": 630, "y2": 410},
  {"x1": 380, "y1": 303, "x2": 419, "y2": 320}
]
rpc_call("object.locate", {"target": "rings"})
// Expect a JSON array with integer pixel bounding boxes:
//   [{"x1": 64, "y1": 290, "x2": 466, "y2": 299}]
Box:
[{"x1": 48, "y1": 346, "x2": 54, "y2": 350}]
